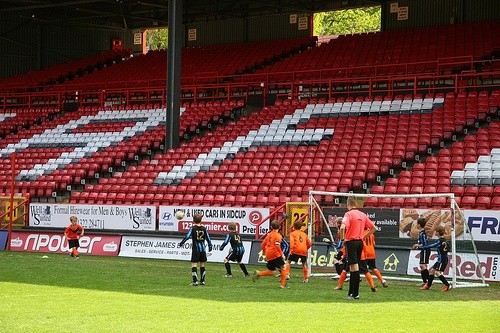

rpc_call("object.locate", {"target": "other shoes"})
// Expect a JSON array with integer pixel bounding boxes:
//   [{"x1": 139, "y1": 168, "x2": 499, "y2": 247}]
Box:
[
  {"x1": 279, "y1": 275, "x2": 290, "y2": 282},
  {"x1": 303, "y1": 279, "x2": 308, "y2": 283},
  {"x1": 200, "y1": 281, "x2": 205, "y2": 285},
  {"x1": 252, "y1": 271, "x2": 258, "y2": 281},
  {"x1": 190, "y1": 282, "x2": 198, "y2": 285},
  {"x1": 334, "y1": 286, "x2": 342, "y2": 290},
  {"x1": 348, "y1": 294, "x2": 359, "y2": 299},
  {"x1": 76, "y1": 256, "x2": 79, "y2": 259},
  {"x1": 280, "y1": 285, "x2": 290, "y2": 289},
  {"x1": 372, "y1": 287, "x2": 378, "y2": 292},
  {"x1": 383, "y1": 282, "x2": 388, "y2": 288},
  {"x1": 224, "y1": 274, "x2": 232, "y2": 278}
]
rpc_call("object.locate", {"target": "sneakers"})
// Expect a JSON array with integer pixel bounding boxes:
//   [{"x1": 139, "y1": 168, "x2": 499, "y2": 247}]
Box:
[
  {"x1": 420, "y1": 283, "x2": 431, "y2": 290},
  {"x1": 442, "y1": 285, "x2": 451, "y2": 291}
]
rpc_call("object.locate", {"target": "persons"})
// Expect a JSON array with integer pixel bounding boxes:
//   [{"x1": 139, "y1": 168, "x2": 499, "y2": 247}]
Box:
[
  {"x1": 413, "y1": 217, "x2": 434, "y2": 287},
  {"x1": 276, "y1": 225, "x2": 290, "y2": 282},
  {"x1": 252, "y1": 220, "x2": 290, "y2": 288},
  {"x1": 285, "y1": 220, "x2": 312, "y2": 283},
  {"x1": 324, "y1": 217, "x2": 389, "y2": 292},
  {"x1": 219, "y1": 222, "x2": 250, "y2": 278},
  {"x1": 340, "y1": 197, "x2": 378, "y2": 299},
  {"x1": 416, "y1": 226, "x2": 452, "y2": 292},
  {"x1": 179, "y1": 213, "x2": 212, "y2": 286},
  {"x1": 64, "y1": 216, "x2": 84, "y2": 260}
]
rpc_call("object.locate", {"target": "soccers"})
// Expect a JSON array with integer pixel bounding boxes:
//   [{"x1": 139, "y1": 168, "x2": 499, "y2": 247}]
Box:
[{"x1": 175, "y1": 210, "x2": 185, "y2": 220}]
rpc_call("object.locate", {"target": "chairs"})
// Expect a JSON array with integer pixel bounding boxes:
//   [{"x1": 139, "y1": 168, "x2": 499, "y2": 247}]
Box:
[{"x1": 0, "y1": 18, "x2": 500, "y2": 211}]
